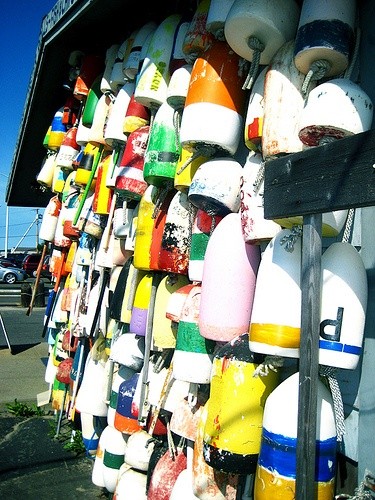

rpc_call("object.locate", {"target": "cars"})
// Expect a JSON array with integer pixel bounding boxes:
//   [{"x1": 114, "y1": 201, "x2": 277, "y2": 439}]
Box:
[
  {"x1": 0, "y1": 264, "x2": 28, "y2": 284},
  {"x1": 0, "y1": 257, "x2": 18, "y2": 268},
  {"x1": 41, "y1": 255, "x2": 54, "y2": 282}
]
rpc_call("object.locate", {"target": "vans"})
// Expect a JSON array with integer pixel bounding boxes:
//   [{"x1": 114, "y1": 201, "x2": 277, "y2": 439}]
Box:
[{"x1": 22, "y1": 254, "x2": 48, "y2": 278}]
